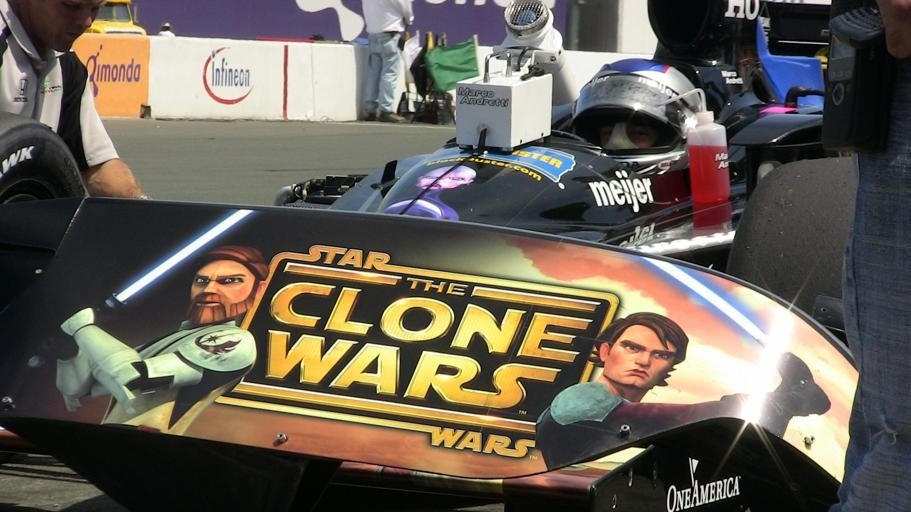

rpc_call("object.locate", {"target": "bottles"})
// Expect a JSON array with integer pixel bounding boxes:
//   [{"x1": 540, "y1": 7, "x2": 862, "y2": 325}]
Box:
[
  {"x1": 694, "y1": 204, "x2": 732, "y2": 240},
  {"x1": 685, "y1": 109, "x2": 731, "y2": 206}
]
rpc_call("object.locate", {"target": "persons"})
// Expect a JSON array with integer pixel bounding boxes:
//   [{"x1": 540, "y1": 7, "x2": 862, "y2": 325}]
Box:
[
  {"x1": 534, "y1": 314, "x2": 831, "y2": 470},
  {"x1": 834, "y1": 0, "x2": 911, "y2": 511},
  {"x1": 1, "y1": 1, "x2": 143, "y2": 200},
  {"x1": 159, "y1": 20, "x2": 176, "y2": 36},
  {"x1": 358, "y1": 0, "x2": 416, "y2": 124},
  {"x1": 34, "y1": 247, "x2": 269, "y2": 439}
]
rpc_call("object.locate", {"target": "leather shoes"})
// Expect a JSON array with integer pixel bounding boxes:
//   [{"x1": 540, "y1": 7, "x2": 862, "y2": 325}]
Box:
[
  {"x1": 362, "y1": 109, "x2": 375, "y2": 121},
  {"x1": 379, "y1": 112, "x2": 408, "y2": 123},
  {"x1": 779, "y1": 347, "x2": 831, "y2": 419}
]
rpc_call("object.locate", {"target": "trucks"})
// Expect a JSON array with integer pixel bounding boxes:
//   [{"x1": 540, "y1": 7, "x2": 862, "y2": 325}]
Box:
[{"x1": 84, "y1": 0, "x2": 146, "y2": 35}]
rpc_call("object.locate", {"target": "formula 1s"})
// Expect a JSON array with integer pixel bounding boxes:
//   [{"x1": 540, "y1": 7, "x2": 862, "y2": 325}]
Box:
[{"x1": 0, "y1": 1, "x2": 858, "y2": 512}]
